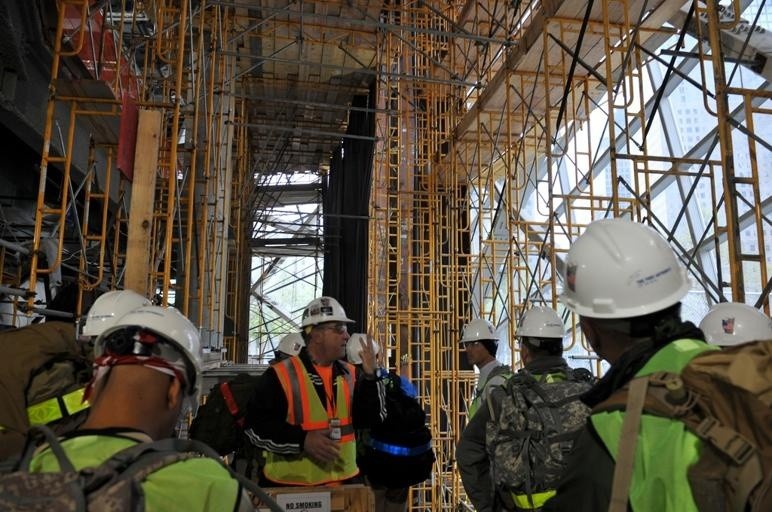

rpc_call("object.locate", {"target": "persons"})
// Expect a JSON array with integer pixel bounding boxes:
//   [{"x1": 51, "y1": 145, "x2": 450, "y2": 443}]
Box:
[
  {"x1": 28, "y1": 288, "x2": 154, "y2": 455},
  {"x1": 697, "y1": 302, "x2": 771, "y2": 359},
  {"x1": 457, "y1": 317, "x2": 514, "y2": 423},
  {"x1": 540, "y1": 215, "x2": 772, "y2": 512},
  {"x1": 229, "y1": 332, "x2": 307, "y2": 505},
  {"x1": 17, "y1": 304, "x2": 258, "y2": 510},
  {"x1": 346, "y1": 328, "x2": 435, "y2": 511},
  {"x1": 454, "y1": 304, "x2": 603, "y2": 511},
  {"x1": 248, "y1": 295, "x2": 375, "y2": 486}
]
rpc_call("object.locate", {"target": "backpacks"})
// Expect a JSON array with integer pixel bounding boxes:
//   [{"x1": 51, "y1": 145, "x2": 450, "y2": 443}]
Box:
[
  {"x1": 1, "y1": 440, "x2": 199, "y2": 511},
  {"x1": 593, "y1": 341, "x2": 772, "y2": 512},
  {"x1": 486, "y1": 367, "x2": 601, "y2": 496},
  {"x1": 359, "y1": 374, "x2": 435, "y2": 493},
  {"x1": 188, "y1": 374, "x2": 261, "y2": 457}
]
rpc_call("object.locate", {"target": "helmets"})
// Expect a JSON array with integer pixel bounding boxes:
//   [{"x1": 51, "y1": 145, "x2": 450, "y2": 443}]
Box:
[
  {"x1": 345, "y1": 334, "x2": 380, "y2": 365},
  {"x1": 513, "y1": 306, "x2": 566, "y2": 339},
  {"x1": 83, "y1": 289, "x2": 152, "y2": 336},
  {"x1": 557, "y1": 218, "x2": 688, "y2": 318},
  {"x1": 698, "y1": 302, "x2": 771, "y2": 347},
  {"x1": 277, "y1": 333, "x2": 305, "y2": 356},
  {"x1": 458, "y1": 318, "x2": 499, "y2": 344},
  {"x1": 93, "y1": 305, "x2": 201, "y2": 417},
  {"x1": 298, "y1": 297, "x2": 355, "y2": 329}
]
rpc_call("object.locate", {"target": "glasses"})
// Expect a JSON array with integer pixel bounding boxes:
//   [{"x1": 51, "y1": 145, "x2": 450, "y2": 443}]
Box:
[{"x1": 314, "y1": 325, "x2": 348, "y2": 335}]
